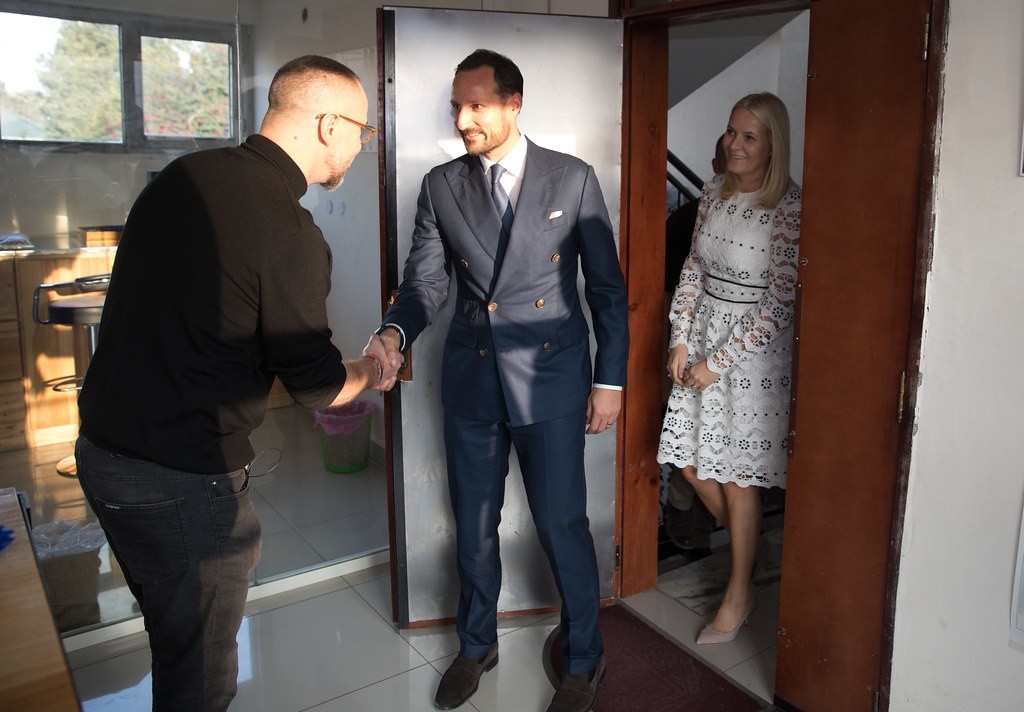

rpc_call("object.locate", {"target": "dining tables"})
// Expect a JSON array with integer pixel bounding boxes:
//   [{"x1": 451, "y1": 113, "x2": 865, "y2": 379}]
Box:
[{"x1": 0, "y1": 486, "x2": 81, "y2": 712}]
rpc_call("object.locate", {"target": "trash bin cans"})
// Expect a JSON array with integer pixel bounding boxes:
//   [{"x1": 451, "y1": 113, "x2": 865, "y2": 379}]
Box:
[{"x1": 314, "y1": 399, "x2": 379, "y2": 473}]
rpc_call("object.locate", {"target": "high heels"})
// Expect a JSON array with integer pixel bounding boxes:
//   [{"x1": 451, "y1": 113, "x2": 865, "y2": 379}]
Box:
[{"x1": 696, "y1": 587, "x2": 755, "y2": 644}]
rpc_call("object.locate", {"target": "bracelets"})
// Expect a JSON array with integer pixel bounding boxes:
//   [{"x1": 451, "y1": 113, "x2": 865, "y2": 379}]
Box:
[{"x1": 366, "y1": 353, "x2": 385, "y2": 386}]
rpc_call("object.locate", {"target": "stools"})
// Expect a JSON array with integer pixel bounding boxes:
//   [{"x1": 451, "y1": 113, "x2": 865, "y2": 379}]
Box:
[{"x1": 32, "y1": 274, "x2": 112, "y2": 477}]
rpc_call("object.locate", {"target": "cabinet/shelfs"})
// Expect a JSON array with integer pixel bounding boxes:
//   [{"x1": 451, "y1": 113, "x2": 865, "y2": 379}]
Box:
[{"x1": 0, "y1": 237, "x2": 118, "y2": 451}]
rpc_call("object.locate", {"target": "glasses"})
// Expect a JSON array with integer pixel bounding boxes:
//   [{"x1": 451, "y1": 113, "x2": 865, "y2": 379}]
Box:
[{"x1": 315, "y1": 112, "x2": 377, "y2": 148}]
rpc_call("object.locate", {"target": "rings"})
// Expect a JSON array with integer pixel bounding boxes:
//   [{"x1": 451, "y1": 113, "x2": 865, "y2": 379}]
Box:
[
  {"x1": 606, "y1": 423, "x2": 612, "y2": 428},
  {"x1": 691, "y1": 385, "x2": 697, "y2": 390},
  {"x1": 667, "y1": 373, "x2": 671, "y2": 377}
]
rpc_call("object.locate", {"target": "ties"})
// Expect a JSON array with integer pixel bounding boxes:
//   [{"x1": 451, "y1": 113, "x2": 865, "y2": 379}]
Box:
[{"x1": 488, "y1": 162, "x2": 514, "y2": 233}]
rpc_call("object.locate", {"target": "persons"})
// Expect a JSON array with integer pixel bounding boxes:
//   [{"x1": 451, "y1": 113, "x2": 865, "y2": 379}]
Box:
[
  {"x1": 370, "y1": 48, "x2": 630, "y2": 712},
  {"x1": 656, "y1": 93, "x2": 804, "y2": 644},
  {"x1": 658, "y1": 134, "x2": 728, "y2": 565},
  {"x1": 73, "y1": 54, "x2": 404, "y2": 712}
]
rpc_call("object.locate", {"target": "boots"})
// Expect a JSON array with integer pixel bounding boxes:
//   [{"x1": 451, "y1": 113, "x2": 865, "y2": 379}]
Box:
[{"x1": 665, "y1": 495, "x2": 713, "y2": 550}]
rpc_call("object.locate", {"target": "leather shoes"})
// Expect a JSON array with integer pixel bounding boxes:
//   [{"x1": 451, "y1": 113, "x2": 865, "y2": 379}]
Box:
[
  {"x1": 546, "y1": 653, "x2": 608, "y2": 712},
  {"x1": 432, "y1": 640, "x2": 498, "y2": 712}
]
